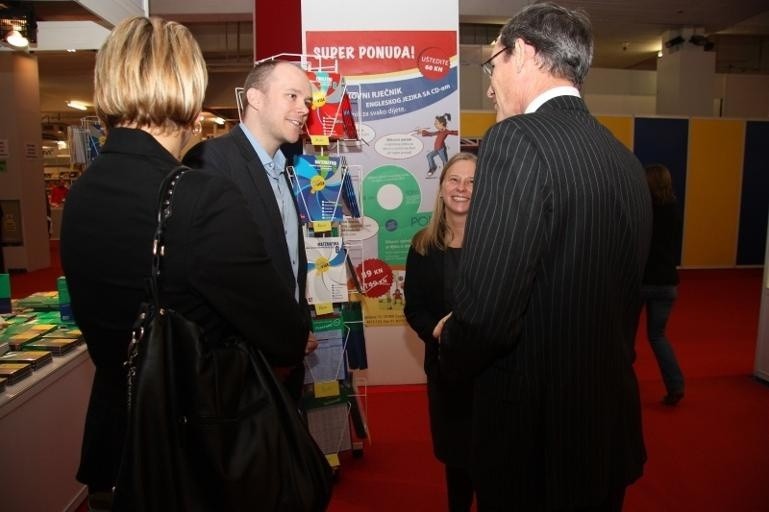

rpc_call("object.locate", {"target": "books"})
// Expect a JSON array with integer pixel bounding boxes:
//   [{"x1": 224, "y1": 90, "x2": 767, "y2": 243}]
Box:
[
  {"x1": 0, "y1": 271, "x2": 109, "y2": 392},
  {"x1": 65, "y1": 121, "x2": 106, "y2": 174},
  {"x1": 283, "y1": 71, "x2": 370, "y2": 457}
]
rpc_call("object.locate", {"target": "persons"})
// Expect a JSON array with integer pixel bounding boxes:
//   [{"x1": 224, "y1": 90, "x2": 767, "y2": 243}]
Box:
[
  {"x1": 180, "y1": 53, "x2": 317, "y2": 436},
  {"x1": 403, "y1": 151, "x2": 495, "y2": 512},
  {"x1": 412, "y1": 113, "x2": 460, "y2": 178},
  {"x1": 643, "y1": 160, "x2": 686, "y2": 407},
  {"x1": 430, "y1": 3, "x2": 653, "y2": 512},
  {"x1": 47, "y1": 177, "x2": 71, "y2": 210},
  {"x1": 61, "y1": 15, "x2": 312, "y2": 510}
]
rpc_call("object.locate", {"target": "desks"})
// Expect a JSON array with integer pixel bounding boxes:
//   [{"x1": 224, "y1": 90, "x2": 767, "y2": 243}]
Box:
[{"x1": 0, "y1": 344, "x2": 99, "y2": 511}]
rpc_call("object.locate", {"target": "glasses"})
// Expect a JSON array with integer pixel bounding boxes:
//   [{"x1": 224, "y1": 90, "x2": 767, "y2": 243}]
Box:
[{"x1": 479, "y1": 44, "x2": 513, "y2": 78}]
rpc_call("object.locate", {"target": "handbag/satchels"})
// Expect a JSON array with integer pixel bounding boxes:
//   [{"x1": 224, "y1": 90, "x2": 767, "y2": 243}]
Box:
[{"x1": 123, "y1": 164, "x2": 336, "y2": 509}]
